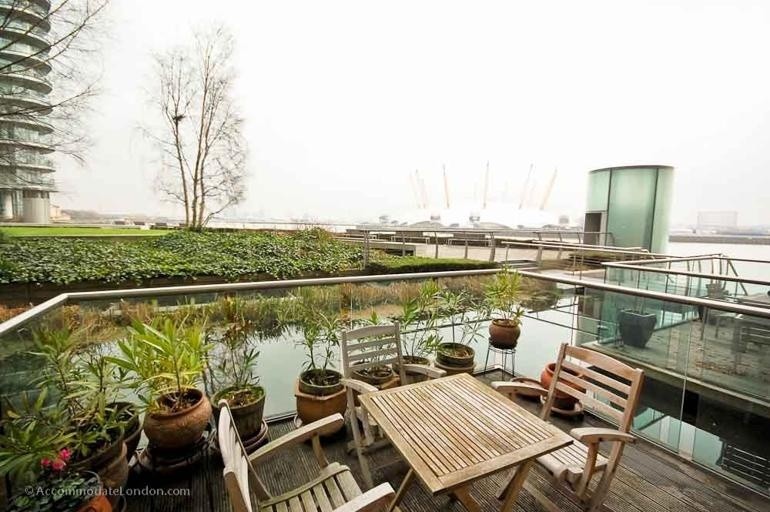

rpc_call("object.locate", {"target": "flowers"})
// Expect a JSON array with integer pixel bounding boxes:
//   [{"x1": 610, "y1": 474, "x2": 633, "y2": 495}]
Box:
[{"x1": 4, "y1": 423, "x2": 82, "y2": 510}]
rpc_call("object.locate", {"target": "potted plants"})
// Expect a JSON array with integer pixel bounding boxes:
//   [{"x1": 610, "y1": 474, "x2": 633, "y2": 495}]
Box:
[
  {"x1": 390, "y1": 279, "x2": 433, "y2": 372},
  {"x1": 2, "y1": 315, "x2": 143, "y2": 493},
  {"x1": 102, "y1": 307, "x2": 212, "y2": 453},
  {"x1": 210, "y1": 320, "x2": 266, "y2": 443},
  {"x1": 617, "y1": 300, "x2": 656, "y2": 348},
  {"x1": 480, "y1": 260, "x2": 528, "y2": 350},
  {"x1": 351, "y1": 307, "x2": 394, "y2": 386},
  {"x1": 434, "y1": 283, "x2": 478, "y2": 374},
  {"x1": 293, "y1": 308, "x2": 347, "y2": 423}
]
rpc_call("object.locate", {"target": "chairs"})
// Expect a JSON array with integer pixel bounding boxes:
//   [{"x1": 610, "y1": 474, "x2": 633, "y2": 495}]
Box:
[
  {"x1": 715, "y1": 438, "x2": 769, "y2": 490},
  {"x1": 734, "y1": 299, "x2": 770, "y2": 368},
  {"x1": 490, "y1": 342, "x2": 645, "y2": 510},
  {"x1": 699, "y1": 282, "x2": 733, "y2": 341},
  {"x1": 217, "y1": 400, "x2": 397, "y2": 512},
  {"x1": 341, "y1": 320, "x2": 408, "y2": 490}
]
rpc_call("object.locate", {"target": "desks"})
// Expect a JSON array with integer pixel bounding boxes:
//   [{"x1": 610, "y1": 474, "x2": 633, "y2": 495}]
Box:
[
  {"x1": 737, "y1": 290, "x2": 770, "y2": 308},
  {"x1": 357, "y1": 371, "x2": 574, "y2": 511}
]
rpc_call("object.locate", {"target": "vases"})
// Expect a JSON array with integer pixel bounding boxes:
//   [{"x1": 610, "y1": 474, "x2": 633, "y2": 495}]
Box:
[{"x1": 85, "y1": 465, "x2": 116, "y2": 512}]
